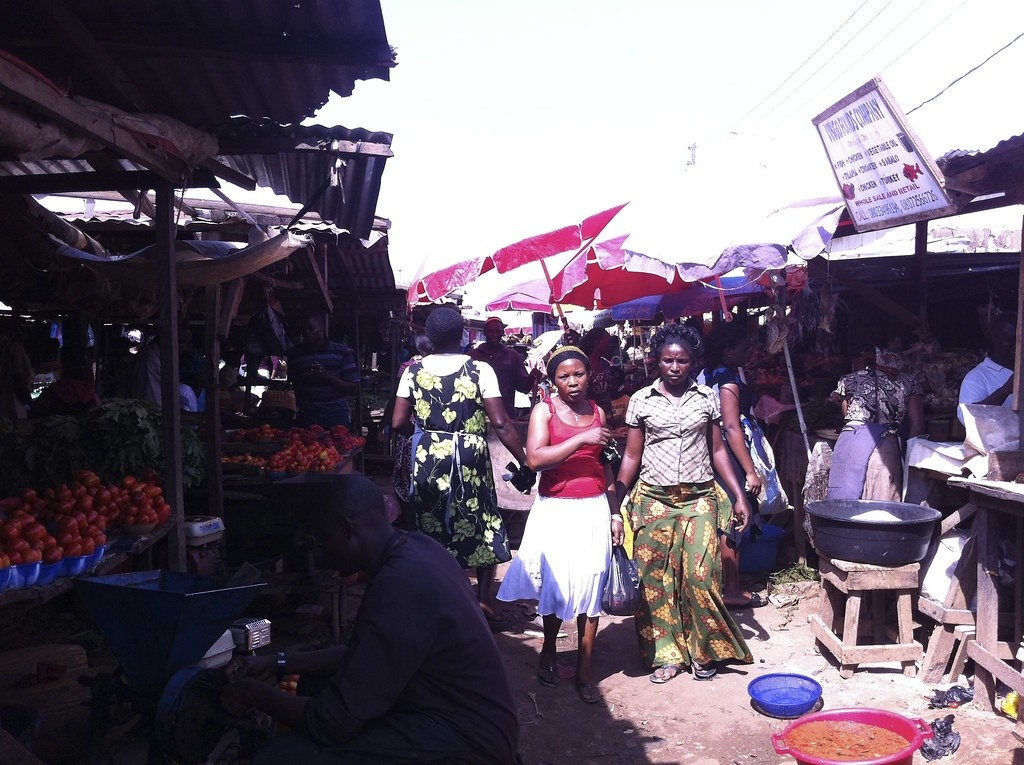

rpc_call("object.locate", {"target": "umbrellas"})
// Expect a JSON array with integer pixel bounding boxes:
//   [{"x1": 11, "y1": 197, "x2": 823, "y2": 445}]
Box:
[{"x1": 405, "y1": 198, "x2": 848, "y2": 463}]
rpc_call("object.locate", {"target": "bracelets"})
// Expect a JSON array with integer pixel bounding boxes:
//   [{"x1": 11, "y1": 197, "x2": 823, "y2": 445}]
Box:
[{"x1": 276, "y1": 651, "x2": 287, "y2": 667}]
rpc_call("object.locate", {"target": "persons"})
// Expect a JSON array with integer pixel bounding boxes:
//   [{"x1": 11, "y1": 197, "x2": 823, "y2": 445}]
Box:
[
  {"x1": 496, "y1": 345, "x2": 625, "y2": 704},
  {"x1": 826, "y1": 347, "x2": 925, "y2": 502},
  {"x1": 467, "y1": 317, "x2": 543, "y2": 421},
  {"x1": 387, "y1": 307, "x2": 527, "y2": 622},
  {"x1": 615, "y1": 322, "x2": 753, "y2": 684},
  {"x1": 277, "y1": 310, "x2": 361, "y2": 431},
  {"x1": 219, "y1": 473, "x2": 520, "y2": 765},
  {"x1": 578, "y1": 327, "x2": 616, "y2": 431},
  {"x1": 696, "y1": 320, "x2": 768, "y2": 610},
  {"x1": 956, "y1": 312, "x2": 1018, "y2": 429}
]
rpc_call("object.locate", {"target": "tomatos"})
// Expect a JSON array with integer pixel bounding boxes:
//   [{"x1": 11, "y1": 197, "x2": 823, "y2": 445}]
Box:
[
  {"x1": 220, "y1": 425, "x2": 366, "y2": 471},
  {"x1": 0, "y1": 470, "x2": 171, "y2": 567}
]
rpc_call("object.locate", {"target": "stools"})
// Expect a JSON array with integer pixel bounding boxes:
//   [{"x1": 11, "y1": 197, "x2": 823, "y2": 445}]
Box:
[{"x1": 807, "y1": 548, "x2": 924, "y2": 679}]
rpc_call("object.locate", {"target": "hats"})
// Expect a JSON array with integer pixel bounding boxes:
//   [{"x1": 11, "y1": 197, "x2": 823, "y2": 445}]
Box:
[{"x1": 549, "y1": 346, "x2": 591, "y2": 385}]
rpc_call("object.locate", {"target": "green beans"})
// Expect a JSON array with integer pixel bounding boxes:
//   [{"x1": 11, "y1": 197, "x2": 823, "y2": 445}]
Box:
[{"x1": 347, "y1": 395, "x2": 386, "y2": 408}]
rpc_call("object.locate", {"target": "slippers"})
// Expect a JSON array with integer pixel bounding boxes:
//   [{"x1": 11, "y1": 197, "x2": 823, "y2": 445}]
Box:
[
  {"x1": 649, "y1": 664, "x2": 718, "y2": 684},
  {"x1": 539, "y1": 659, "x2": 562, "y2": 688},
  {"x1": 723, "y1": 591, "x2": 768, "y2": 608},
  {"x1": 576, "y1": 680, "x2": 603, "y2": 704}
]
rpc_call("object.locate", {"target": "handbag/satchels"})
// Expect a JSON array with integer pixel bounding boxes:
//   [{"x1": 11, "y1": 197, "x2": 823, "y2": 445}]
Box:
[{"x1": 602, "y1": 544, "x2": 645, "y2": 616}]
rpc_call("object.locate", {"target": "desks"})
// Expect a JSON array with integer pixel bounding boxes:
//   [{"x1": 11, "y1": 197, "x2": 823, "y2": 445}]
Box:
[
  {"x1": 0, "y1": 512, "x2": 178, "y2": 620},
  {"x1": 922, "y1": 468, "x2": 1024, "y2": 712}
]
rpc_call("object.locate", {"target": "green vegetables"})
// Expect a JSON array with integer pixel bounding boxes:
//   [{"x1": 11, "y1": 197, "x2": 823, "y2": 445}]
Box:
[{"x1": 42, "y1": 399, "x2": 208, "y2": 488}]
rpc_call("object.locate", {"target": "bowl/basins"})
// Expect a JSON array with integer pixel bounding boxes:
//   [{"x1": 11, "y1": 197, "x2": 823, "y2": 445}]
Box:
[
  {"x1": 770, "y1": 707, "x2": 932, "y2": 765},
  {"x1": 801, "y1": 499, "x2": 943, "y2": 566}
]
